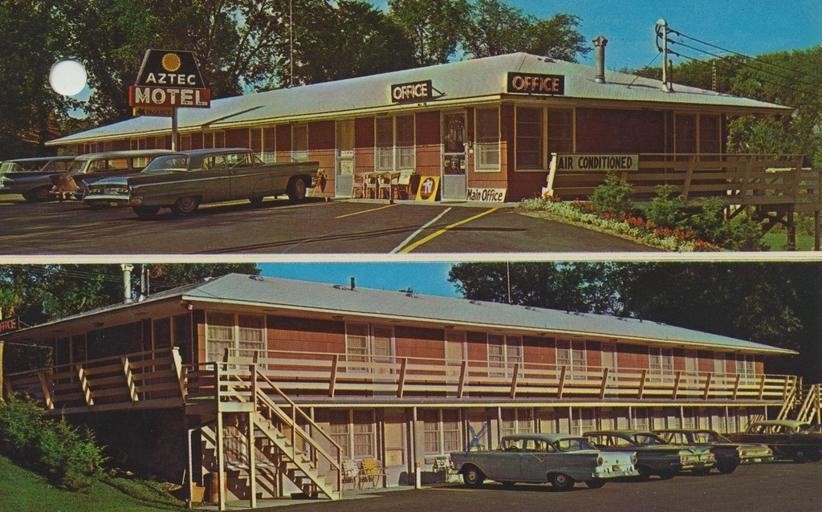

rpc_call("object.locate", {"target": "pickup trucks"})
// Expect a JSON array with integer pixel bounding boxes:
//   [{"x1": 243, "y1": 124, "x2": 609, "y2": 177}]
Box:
[{"x1": 0, "y1": 154, "x2": 75, "y2": 202}]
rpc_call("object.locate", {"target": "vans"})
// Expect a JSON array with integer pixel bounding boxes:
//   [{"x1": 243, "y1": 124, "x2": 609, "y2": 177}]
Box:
[
  {"x1": 0, "y1": 157, "x2": 48, "y2": 190},
  {"x1": 49, "y1": 148, "x2": 177, "y2": 208}
]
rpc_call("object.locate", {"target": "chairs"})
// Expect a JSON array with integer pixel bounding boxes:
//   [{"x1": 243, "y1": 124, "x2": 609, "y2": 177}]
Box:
[
  {"x1": 434, "y1": 455, "x2": 459, "y2": 480},
  {"x1": 352, "y1": 169, "x2": 411, "y2": 200},
  {"x1": 344, "y1": 458, "x2": 387, "y2": 489}
]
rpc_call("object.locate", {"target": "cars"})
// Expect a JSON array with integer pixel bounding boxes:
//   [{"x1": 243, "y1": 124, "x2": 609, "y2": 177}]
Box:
[
  {"x1": 85, "y1": 144, "x2": 320, "y2": 218},
  {"x1": 720, "y1": 420, "x2": 822, "y2": 466},
  {"x1": 449, "y1": 433, "x2": 640, "y2": 490},
  {"x1": 652, "y1": 429, "x2": 774, "y2": 476},
  {"x1": 579, "y1": 428, "x2": 716, "y2": 480}
]
